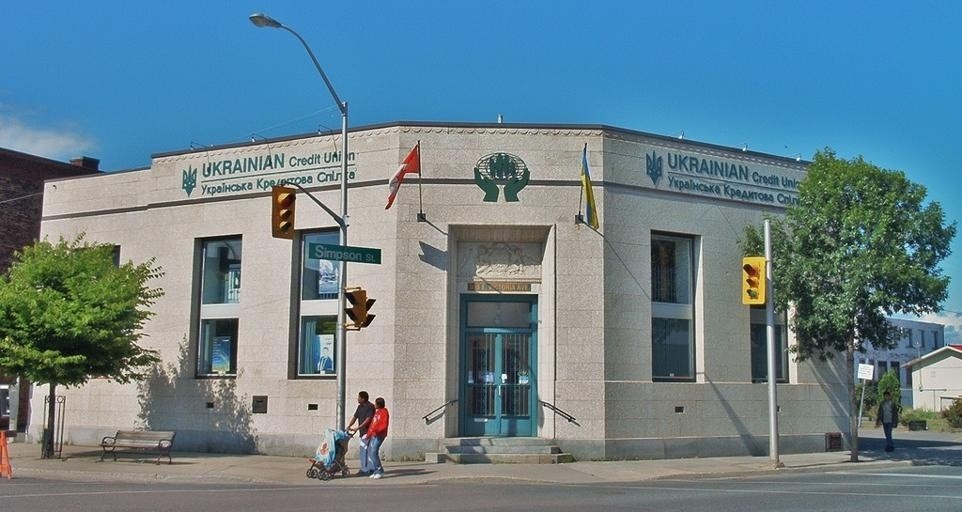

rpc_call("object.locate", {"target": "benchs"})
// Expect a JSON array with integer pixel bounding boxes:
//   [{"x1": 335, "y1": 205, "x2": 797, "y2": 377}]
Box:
[{"x1": 95, "y1": 429, "x2": 178, "y2": 465}]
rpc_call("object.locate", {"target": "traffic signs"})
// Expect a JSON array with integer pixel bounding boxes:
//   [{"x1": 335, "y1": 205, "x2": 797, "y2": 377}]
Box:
[{"x1": 307, "y1": 241, "x2": 382, "y2": 267}]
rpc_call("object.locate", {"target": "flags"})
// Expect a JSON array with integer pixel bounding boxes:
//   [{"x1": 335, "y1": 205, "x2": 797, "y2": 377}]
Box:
[
  {"x1": 385, "y1": 144, "x2": 419, "y2": 210},
  {"x1": 581, "y1": 150, "x2": 600, "y2": 230}
]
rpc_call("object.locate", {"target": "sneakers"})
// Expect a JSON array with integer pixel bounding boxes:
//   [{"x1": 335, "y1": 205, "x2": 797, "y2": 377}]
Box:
[{"x1": 369, "y1": 474, "x2": 381, "y2": 479}]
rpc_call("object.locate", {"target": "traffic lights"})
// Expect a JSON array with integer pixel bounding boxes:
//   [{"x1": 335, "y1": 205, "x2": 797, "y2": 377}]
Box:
[
  {"x1": 270, "y1": 183, "x2": 296, "y2": 242},
  {"x1": 743, "y1": 256, "x2": 766, "y2": 304},
  {"x1": 342, "y1": 288, "x2": 368, "y2": 325}
]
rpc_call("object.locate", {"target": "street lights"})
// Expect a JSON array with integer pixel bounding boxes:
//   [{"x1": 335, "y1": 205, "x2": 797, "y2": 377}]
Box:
[{"x1": 248, "y1": 11, "x2": 347, "y2": 433}]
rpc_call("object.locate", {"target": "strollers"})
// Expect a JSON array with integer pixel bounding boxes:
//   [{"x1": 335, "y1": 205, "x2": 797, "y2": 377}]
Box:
[{"x1": 306, "y1": 426, "x2": 357, "y2": 482}]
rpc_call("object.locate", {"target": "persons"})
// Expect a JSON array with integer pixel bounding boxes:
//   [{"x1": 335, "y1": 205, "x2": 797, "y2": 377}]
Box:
[
  {"x1": 318, "y1": 346, "x2": 332, "y2": 370},
  {"x1": 345, "y1": 390, "x2": 376, "y2": 475},
  {"x1": 362, "y1": 396, "x2": 389, "y2": 480},
  {"x1": 875, "y1": 391, "x2": 899, "y2": 453}
]
